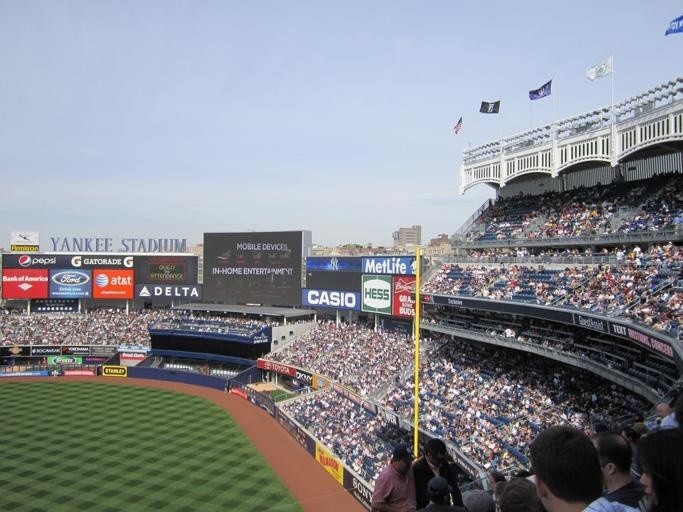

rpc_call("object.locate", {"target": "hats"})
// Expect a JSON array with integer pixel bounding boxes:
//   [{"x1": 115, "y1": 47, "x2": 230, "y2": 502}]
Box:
[
  {"x1": 428, "y1": 476, "x2": 452, "y2": 495},
  {"x1": 393, "y1": 444, "x2": 416, "y2": 462},
  {"x1": 461, "y1": 489, "x2": 495, "y2": 512}
]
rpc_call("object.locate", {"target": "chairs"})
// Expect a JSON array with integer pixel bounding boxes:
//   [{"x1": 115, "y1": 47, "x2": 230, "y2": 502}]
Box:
[
  {"x1": 434, "y1": 181, "x2": 682, "y2": 319},
  {"x1": 270, "y1": 321, "x2": 680, "y2": 491}
]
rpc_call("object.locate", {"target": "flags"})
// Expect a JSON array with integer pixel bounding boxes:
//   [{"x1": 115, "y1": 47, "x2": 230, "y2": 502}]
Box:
[
  {"x1": 529, "y1": 81, "x2": 551, "y2": 100},
  {"x1": 479, "y1": 101, "x2": 500, "y2": 113},
  {"x1": 665, "y1": 15, "x2": 683, "y2": 35},
  {"x1": 453, "y1": 117, "x2": 462, "y2": 135},
  {"x1": 585, "y1": 56, "x2": 613, "y2": 80}
]
